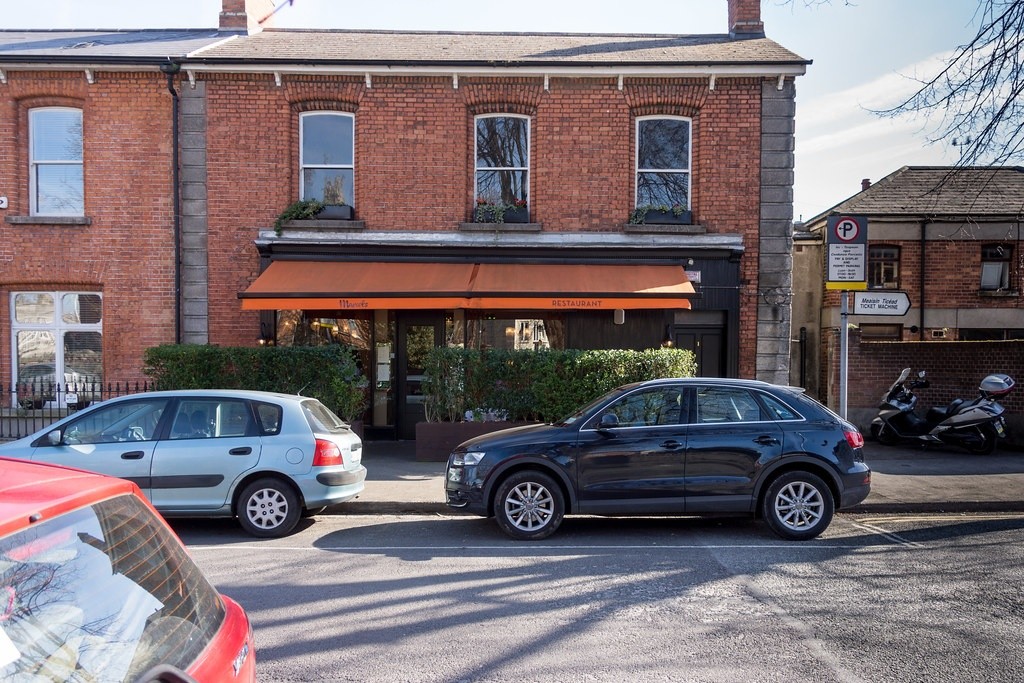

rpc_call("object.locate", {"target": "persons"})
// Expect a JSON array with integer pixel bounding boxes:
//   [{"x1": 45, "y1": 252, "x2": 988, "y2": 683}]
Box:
[{"x1": 174, "y1": 414, "x2": 193, "y2": 438}]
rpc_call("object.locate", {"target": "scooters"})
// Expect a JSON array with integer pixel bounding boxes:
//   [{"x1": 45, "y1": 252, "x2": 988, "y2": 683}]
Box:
[{"x1": 870, "y1": 368, "x2": 1017, "y2": 455}]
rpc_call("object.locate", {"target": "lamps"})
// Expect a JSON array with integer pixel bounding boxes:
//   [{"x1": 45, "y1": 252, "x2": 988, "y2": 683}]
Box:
[
  {"x1": 255, "y1": 322, "x2": 267, "y2": 346},
  {"x1": 664, "y1": 324, "x2": 672, "y2": 347}
]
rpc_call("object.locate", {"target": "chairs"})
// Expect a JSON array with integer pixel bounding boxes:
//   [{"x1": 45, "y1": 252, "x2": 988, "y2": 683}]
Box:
[
  {"x1": 190, "y1": 410, "x2": 208, "y2": 437},
  {"x1": 174, "y1": 413, "x2": 192, "y2": 438},
  {"x1": 666, "y1": 396, "x2": 681, "y2": 425}
]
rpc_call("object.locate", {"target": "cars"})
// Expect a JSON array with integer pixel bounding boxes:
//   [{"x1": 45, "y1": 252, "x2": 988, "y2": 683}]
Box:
[
  {"x1": 0, "y1": 388, "x2": 367, "y2": 539},
  {"x1": 444, "y1": 377, "x2": 873, "y2": 541},
  {"x1": 1, "y1": 457, "x2": 257, "y2": 683},
  {"x1": 17, "y1": 361, "x2": 103, "y2": 410}
]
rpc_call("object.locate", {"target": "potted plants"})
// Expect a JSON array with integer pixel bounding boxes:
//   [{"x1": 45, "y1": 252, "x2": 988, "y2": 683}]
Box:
[
  {"x1": 273, "y1": 198, "x2": 354, "y2": 237},
  {"x1": 68, "y1": 397, "x2": 90, "y2": 410},
  {"x1": 16, "y1": 396, "x2": 46, "y2": 417},
  {"x1": 630, "y1": 203, "x2": 692, "y2": 226}
]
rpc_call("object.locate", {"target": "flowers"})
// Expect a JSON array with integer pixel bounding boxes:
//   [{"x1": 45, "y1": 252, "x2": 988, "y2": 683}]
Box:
[{"x1": 476, "y1": 198, "x2": 527, "y2": 242}]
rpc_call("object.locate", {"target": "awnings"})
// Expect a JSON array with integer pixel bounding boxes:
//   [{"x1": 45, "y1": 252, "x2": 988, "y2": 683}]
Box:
[{"x1": 238, "y1": 261, "x2": 703, "y2": 310}]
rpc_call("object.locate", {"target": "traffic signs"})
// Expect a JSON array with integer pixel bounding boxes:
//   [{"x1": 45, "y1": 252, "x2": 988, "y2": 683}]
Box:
[{"x1": 853, "y1": 291, "x2": 912, "y2": 316}]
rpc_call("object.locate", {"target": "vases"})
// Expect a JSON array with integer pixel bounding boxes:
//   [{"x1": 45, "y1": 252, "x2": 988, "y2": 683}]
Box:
[{"x1": 474, "y1": 207, "x2": 529, "y2": 223}]
